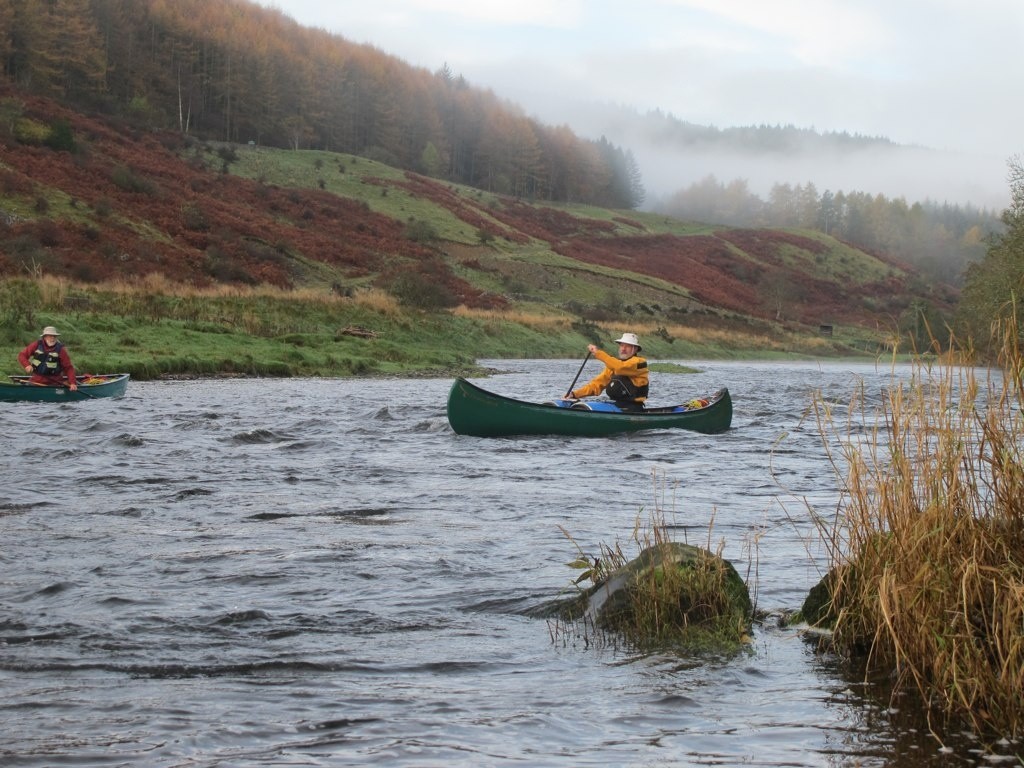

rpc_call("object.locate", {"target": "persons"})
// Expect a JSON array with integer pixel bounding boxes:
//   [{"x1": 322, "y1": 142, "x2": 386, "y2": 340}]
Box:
[
  {"x1": 562, "y1": 332, "x2": 649, "y2": 413},
  {"x1": 18, "y1": 326, "x2": 77, "y2": 391}
]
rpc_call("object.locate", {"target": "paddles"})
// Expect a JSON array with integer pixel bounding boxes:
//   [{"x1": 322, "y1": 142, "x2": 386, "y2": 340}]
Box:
[
  {"x1": 564, "y1": 348, "x2": 593, "y2": 398},
  {"x1": 32, "y1": 370, "x2": 97, "y2": 399}
]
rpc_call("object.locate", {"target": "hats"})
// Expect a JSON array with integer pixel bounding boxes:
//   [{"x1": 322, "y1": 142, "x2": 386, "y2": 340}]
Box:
[
  {"x1": 615, "y1": 333, "x2": 642, "y2": 352},
  {"x1": 40, "y1": 326, "x2": 61, "y2": 337}
]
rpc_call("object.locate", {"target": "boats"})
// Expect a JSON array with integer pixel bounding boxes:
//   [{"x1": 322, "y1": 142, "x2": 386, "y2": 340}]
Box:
[
  {"x1": 444, "y1": 374, "x2": 734, "y2": 439},
  {"x1": 0, "y1": 373, "x2": 131, "y2": 403}
]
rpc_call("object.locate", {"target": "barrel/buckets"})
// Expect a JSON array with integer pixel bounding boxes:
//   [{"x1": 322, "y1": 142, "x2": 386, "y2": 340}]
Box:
[
  {"x1": 569, "y1": 402, "x2": 623, "y2": 412},
  {"x1": 542, "y1": 400, "x2": 573, "y2": 408}
]
